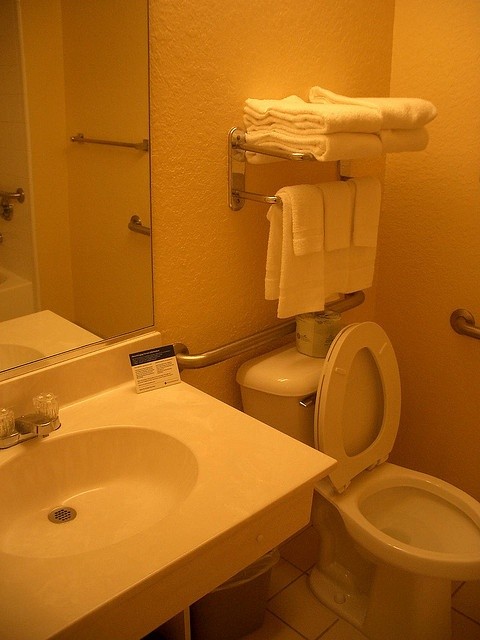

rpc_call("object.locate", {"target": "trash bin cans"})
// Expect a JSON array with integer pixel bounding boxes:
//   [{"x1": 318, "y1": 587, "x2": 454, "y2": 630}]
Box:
[{"x1": 190, "y1": 548, "x2": 280, "y2": 640}]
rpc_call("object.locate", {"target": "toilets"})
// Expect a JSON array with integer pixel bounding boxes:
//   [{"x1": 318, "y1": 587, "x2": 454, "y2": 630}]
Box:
[{"x1": 235, "y1": 322, "x2": 478, "y2": 637}]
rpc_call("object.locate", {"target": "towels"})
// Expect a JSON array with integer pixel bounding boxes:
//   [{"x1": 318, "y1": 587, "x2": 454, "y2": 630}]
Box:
[
  {"x1": 266, "y1": 186, "x2": 326, "y2": 256},
  {"x1": 317, "y1": 188, "x2": 353, "y2": 304},
  {"x1": 377, "y1": 128, "x2": 431, "y2": 153},
  {"x1": 309, "y1": 86, "x2": 439, "y2": 129},
  {"x1": 243, "y1": 95, "x2": 382, "y2": 135},
  {"x1": 244, "y1": 132, "x2": 383, "y2": 164},
  {"x1": 345, "y1": 184, "x2": 382, "y2": 294},
  {"x1": 316, "y1": 181, "x2": 351, "y2": 252},
  {"x1": 349, "y1": 176, "x2": 381, "y2": 247}
]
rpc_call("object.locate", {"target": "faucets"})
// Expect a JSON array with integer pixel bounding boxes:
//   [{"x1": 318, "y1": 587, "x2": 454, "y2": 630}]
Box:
[{"x1": 1, "y1": 389, "x2": 64, "y2": 451}]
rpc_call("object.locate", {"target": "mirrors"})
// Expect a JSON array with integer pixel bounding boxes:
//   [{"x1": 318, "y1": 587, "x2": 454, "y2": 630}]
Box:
[{"x1": 0, "y1": 0, "x2": 152, "y2": 373}]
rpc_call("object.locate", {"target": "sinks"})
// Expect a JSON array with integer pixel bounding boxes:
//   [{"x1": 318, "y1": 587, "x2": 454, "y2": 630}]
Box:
[{"x1": 0, "y1": 330, "x2": 198, "y2": 562}]
